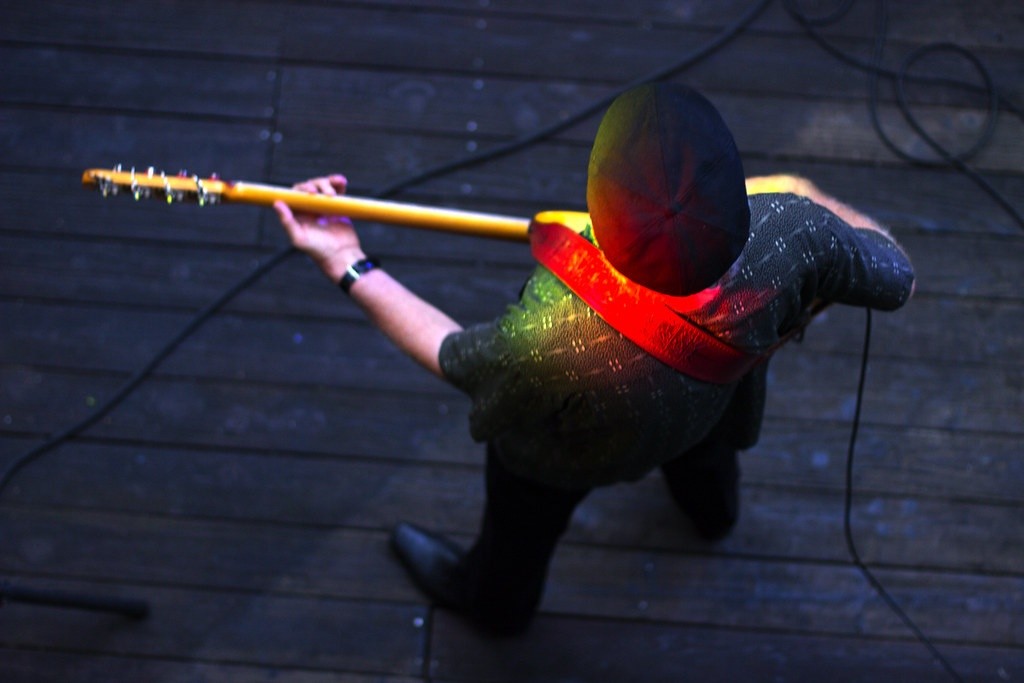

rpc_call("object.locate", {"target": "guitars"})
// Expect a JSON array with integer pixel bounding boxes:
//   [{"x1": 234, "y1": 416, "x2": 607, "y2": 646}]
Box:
[{"x1": 72, "y1": 157, "x2": 830, "y2": 257}]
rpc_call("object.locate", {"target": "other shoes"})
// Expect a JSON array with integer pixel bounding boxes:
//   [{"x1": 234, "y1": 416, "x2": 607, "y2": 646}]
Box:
[{"x1": 389, "y1": 519, "x2": 462, "y2": 593}]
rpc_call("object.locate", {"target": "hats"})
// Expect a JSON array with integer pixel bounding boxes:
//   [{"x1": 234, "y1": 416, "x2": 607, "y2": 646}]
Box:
[{"x1": 585, "y1": 79, "x2": 752, "y2": 296}]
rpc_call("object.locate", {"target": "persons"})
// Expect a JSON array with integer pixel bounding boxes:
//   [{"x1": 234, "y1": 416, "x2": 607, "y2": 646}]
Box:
[{"x1": 274, "y1": 84, "x2": 917, "y2": 641}]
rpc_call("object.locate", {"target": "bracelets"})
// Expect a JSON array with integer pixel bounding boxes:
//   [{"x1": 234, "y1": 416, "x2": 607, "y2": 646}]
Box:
[{"x1": 336, "y1": 255, "x2": 380, "y2": 295}]
